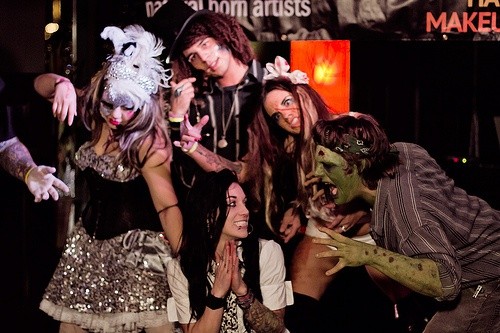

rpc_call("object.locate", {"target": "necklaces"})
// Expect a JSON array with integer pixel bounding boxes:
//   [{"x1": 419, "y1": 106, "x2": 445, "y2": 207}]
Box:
[{"x1": 218, "y1": 89, "x2": 235, "y2": 148}]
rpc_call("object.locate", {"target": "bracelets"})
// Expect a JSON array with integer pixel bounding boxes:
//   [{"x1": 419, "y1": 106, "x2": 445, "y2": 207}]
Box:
[
  {"x1": 169, "y1": 111, "x2": 184, "y2": 128},
  {"x1": 207, "y1": 292, "x2": 225, "y2": 309},
  {"x1": 25, "y1": 166, "x2": 34, "y2": 183},
  {"x1": 186, "y1": 142, "x2": 198, "y2": 155}
]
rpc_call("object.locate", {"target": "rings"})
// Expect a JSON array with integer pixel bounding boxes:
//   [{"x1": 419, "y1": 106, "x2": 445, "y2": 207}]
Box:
[
  {"x1": 173, "y1": 87, "x2": 181, "y2": 97},
  {"x1": 215, "y1": 262, "x2": 219, "y2": 266}
]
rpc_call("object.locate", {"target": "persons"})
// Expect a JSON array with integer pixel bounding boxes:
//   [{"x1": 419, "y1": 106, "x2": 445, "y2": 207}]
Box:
[
  {"x1": 1, "y1": 51, "x2": 71, "y2": 202},
  {"x1": 35, "y1": 25, "x2": 182, "y2": 333},
  {"x1": 152, "y1": 0, "x2": 268, "y2": 239},
  {"x1": 168, "y1": 167, "x2": 294, "y2": 333},
  {"x1": 303, "y1": 112, "x2": 500, "y2": 333},
  {"x1": 173, "y1": 55, "x2": 418, "y2": 333}
]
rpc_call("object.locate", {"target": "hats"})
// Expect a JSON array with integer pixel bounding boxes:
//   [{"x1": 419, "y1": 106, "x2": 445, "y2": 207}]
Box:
[{"x1": 152, "y1": 0, "x2": 199, "y2": 64}]
